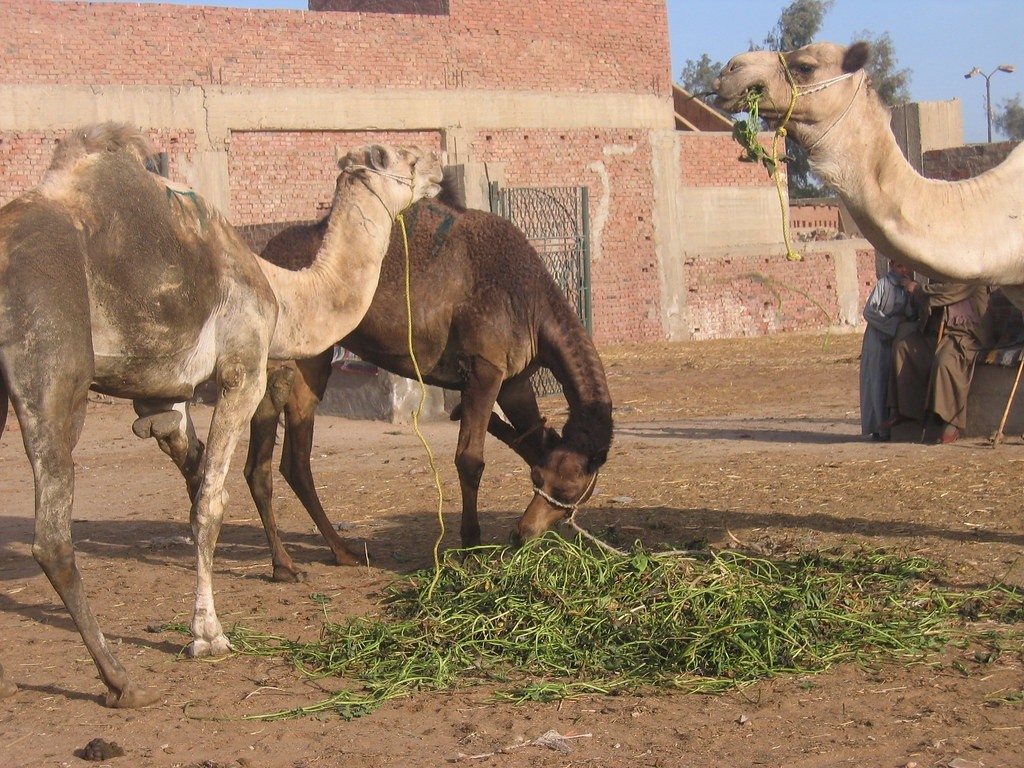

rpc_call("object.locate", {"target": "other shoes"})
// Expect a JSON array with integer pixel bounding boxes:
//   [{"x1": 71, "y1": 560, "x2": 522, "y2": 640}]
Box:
[
  {"x1": 937, "y1": 428, "x2": 960, "y2": 444},
  {"x1": 879, "y1": 415, "x2": 903, "y2": 430}
]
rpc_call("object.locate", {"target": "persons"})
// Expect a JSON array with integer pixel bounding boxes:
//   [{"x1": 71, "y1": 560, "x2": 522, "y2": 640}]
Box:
[
  {"x1": 859, "y1": 259, "x2": 916, "y2": 441},
  {"x1": 880, "y1": 272, "x2": 1000, "y2": 445}
]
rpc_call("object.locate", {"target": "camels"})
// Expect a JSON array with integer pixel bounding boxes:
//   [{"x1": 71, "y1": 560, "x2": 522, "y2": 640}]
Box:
[
  {"x1": 714, "y1": 41, "x2": 1024, "y2": 286},
  {"x1": 1, "y1": 118, "x2": 456, "y2": 709},
  {"x1": 241, "y1": 197, "x2": 615, "y2": 587}
]
